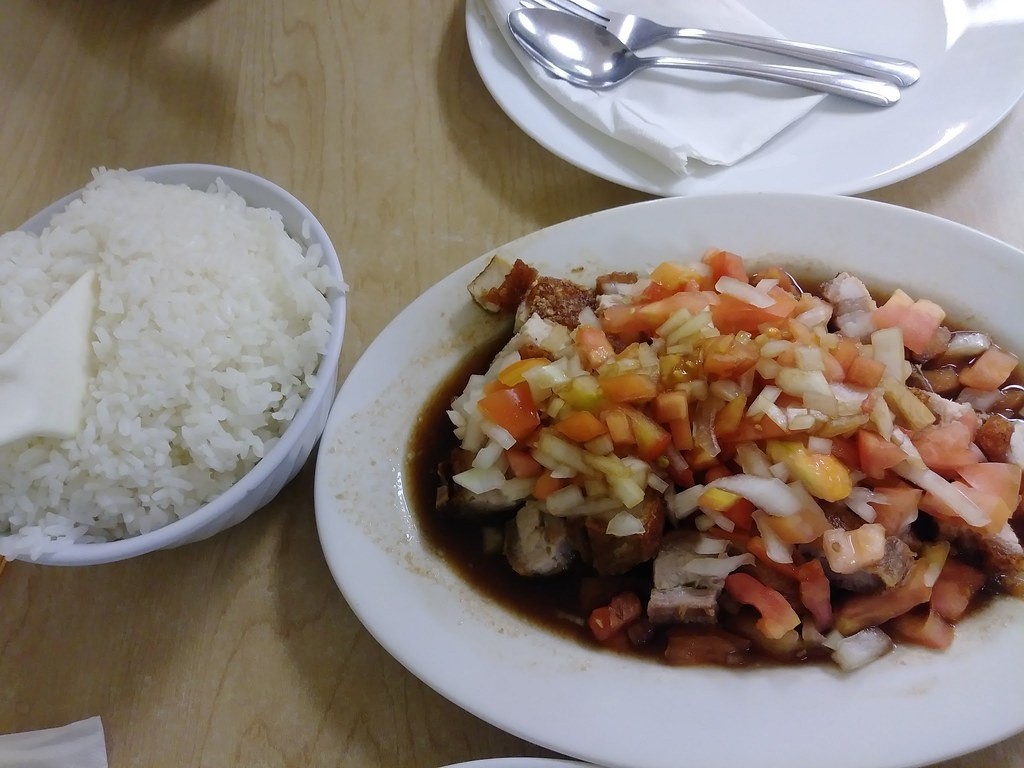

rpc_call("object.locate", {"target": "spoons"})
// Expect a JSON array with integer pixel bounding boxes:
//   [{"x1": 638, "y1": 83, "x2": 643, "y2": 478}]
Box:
[{"x1": 505, "y1": 8, "x2": 901, "y2": 108}]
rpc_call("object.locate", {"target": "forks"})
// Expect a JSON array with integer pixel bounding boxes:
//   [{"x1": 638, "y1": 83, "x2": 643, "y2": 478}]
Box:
[{"x1": 521, "y1": 0, "x2": 921, "y2": 86}]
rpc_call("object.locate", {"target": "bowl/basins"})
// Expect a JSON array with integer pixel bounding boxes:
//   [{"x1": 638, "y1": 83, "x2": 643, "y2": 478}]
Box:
[{"x1": 1, "y1": 155, "x2": 350, "y2": 566}]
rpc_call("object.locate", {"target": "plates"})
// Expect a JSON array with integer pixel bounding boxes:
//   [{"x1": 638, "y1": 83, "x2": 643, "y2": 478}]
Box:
[
  {"x1": 316, "y1": 189, "x2": 1024, "y2": 768},
  {"x1": 462, "y1": 1, "x2": 1023, "y2": 203}
]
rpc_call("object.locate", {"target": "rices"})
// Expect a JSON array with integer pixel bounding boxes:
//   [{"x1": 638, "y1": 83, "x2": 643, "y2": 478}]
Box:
[{"x1": 0, "y1": 164, "x2": 340, "y2": 561}]
